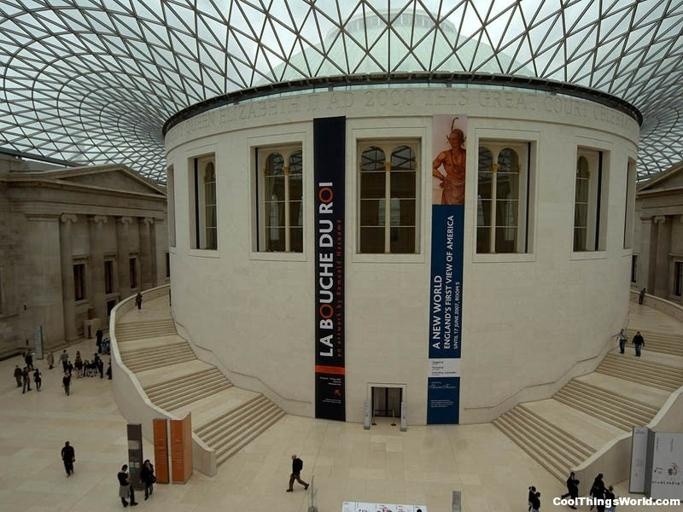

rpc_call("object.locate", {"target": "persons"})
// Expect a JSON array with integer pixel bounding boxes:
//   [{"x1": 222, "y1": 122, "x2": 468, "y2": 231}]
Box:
[
  {"x1": 528, "y1": 471, "x2": 616, "y2": 512},
  {"x1": 136, "y1": 292, "x2": 143, "y2": 310},
  {"x1": 14, "y1": 328, "x2": 111, "y2": 396},
  {"x1": 615, "y1": 329, "x2": 628, "y2": 354},
  {"x1": 638, "y1": 288, "x2": 646, "y2": 304},
  {"x1": 140, "y1": 461, "x2": 156, "y2": 500},
  {"x1": 432, "y1": 129, "x2": 466, "y2": 204},
  {"x1": 632, "y1": 331, "x2": 645, "y2": 356},
  {"x1": 286, "y1": 455, "x2": 310, "y2": 491},
  {"x1": 61, "y1": 441, "x2": 75, "y2": 476},
  {"x1": 118, "y1": 465, "x2": 138, "y2": 507}
]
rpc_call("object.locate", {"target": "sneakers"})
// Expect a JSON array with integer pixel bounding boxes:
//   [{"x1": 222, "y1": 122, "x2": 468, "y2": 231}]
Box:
[
  {"x1": 286, "y1": 489, "x2": 293, "y2": 492},
  {"x1": 304, "y1": 484, "x2": 309, "y2": 490},
  {"x1": 125, "y1": 497, "x2": 148, "y2": 506}
]
